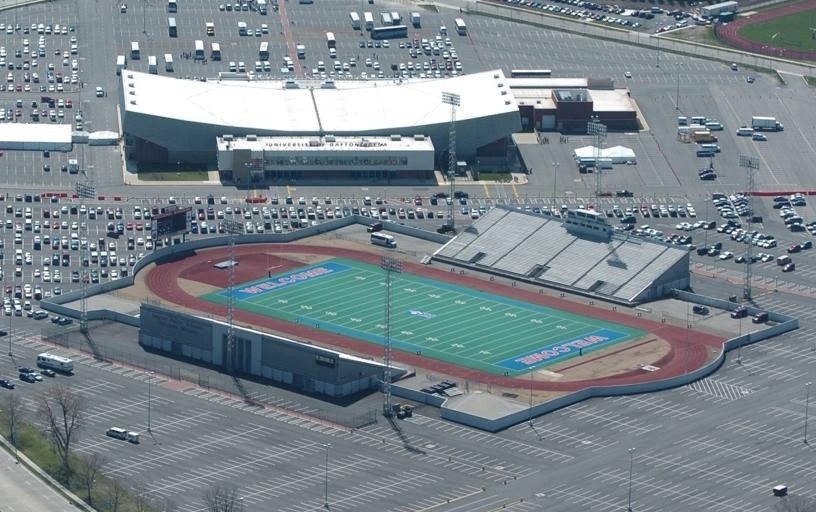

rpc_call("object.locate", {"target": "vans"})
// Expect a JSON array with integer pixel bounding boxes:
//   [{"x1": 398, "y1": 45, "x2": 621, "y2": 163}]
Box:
[
  {"x1": 699, "y1": 170, "x2": 718, "y2": 180},
  {"x1": 420, "y1": 380, "x2": 456, "y2": 395},
  {"x1": 678, "y1": 116, "x2": 724, "y2": 158},
  {"x1": 731, "y1": 62, "x2": 737, "y2": 69},
  {"x1": 44, "y1": 150, "x2": 50, "y2": 157},
  {"x1": 693, "y1": 220, "x2": 714, "y2": 229},
  {"x1": 753, "y1": 311, "x2": 767, "y2": 324},
  {"x1": 730, "y1": 308, "x2": 746, "y2": 318},
  {"x1": 693, "y1": 305, "x2": 709, "y2": 315},
  {"x1": 736, "y1": 128, "x2": 768, "y2": 141}
]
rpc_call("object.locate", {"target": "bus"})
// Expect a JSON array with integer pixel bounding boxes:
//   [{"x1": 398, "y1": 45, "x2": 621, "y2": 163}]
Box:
[
  {"x1": 701, "y1": 1, "x2": 739, "y2": 17},
  {"x1": 347, "y1": 12, "x2": 421, "y2": 39},
  {"x1": 37, "y1": 353, "x2": 73, "y2": 373},
  {"x1": 69, "y1": 159, "x2": 78, "y2": 174},
  {"x1": 454, "y1": 18, "x2": 467, "y2": 35},
  {"x1": 326, "y1": 32, "x2": 336, "y2": 48},
  {"x1": 115, "y1": 0, "x2": 222, "y2": 76}
]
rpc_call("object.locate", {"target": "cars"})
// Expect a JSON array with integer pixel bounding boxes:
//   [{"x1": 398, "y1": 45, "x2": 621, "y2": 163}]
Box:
[
  {"x1": 219, "y1": 0, "x2": 279, "y2": 37},
  {"x1": 0, "y1": 24, "x2": 83, "y2": 130},
  {"x1": 697, "y1": 241, "x2": 747, "y2": 263},
  {"x1": 344, "y1": 192, "x2": 486, "y2": 224},
  {"x1": 712, "y1": 191, "x2": 753, "y2": 217},
  {"x1": 623, "y1": 222, "x2": 696, "y2": 250},
  {"x1": 312, "y1": 36, "x2": 463, "y2": 81},
  {"x1": 43, "y1": 164, "x2": 50, "y2": 171},
  {"x1": 625, "y1": 71, "x2": 632, "y2": 78},
  {"x1": 1, "y1": 367, "x2": 56, "y2": 390},
  {"x1": 748, "y1": 76, "x2": 753, "y2": 83},
  {"x1": 597, "y1": 190, "x2": 634, "y2": 197},
  {"x1": 62, "y1": 164, "x2": 67, "y2": 171},
  {"x1": 506, "y1": 0, "x2": 708, "y2": 35},
  {"x1": 191, "y1": 195, "x2": 343, "y2": 233},
  {"x1": 755, "y1": 241, "x2": 812, "y2": 272},
  {"x1": 2, "y1": 284, "x2": 72, "y2": 326},
  {"x1": 0, "y1": 193, "x2": 158, "y2": 285},
  {"x1": 719, "y1": 219, "x2": 777, "y2": 250},
  {"x1": 515, "y1": 204, "x2": 595, "y2": 219},
  {"x1": 606, "y1": 202, "x2": 697, "y2": 222},
  {"x1": 774, "y1": 193, "x2": 816, "y2": 238}
]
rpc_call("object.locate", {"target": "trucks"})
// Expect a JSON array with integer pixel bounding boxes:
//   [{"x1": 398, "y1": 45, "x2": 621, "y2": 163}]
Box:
[
  {"x1": 752, "y1": 117, "x2": 784, "y2": 131},
  {"x1": 229, "y1": 41, "x2": 306, "y2": 72},
  {"x1": 106, "y1": 427, "x2": 140, "y2": 444},
  {"x1": 367, "y1": 222, "x2": 381, "y2": 233},
  {"x1": 96, "y1": 86, "x2": 104, "y2": 97},
  {"x1": 440, "y1": 26, "x2": 447, "y2": 37},
  {"x1": 370, "y1": 233, "x2": 397, "y2": 248}
]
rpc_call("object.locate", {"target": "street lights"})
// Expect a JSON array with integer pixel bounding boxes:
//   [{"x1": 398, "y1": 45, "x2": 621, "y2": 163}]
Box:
[
  {"x1": 380, "y1": 257, "x2": 405, "y2": 417},
  {"x1": 675, "y1": 62, "x2": 683, "y2": 111},
  {"x1": 222, "y1": 217, "x2": 244, "y2": 375},
  {"x1": 8, "y1": 286, "x2": 17, "y2": 357},
  {"x1": 551, "y1": 162, "x2": 562, "y2": 206},
  {"x1": 323, "y1": 443, "x2": 332, "y2": 506},
  {"x1": 805, "y1": 382, "x2": 812, "y2": 443},
  {"x1": 529, "y1": 365, "x2": 537, "y2": 426},
  {"x1": 703, "y1": 198, "x2": 712, "y2": 273},
  {"x1": 629, "y1": 447, "x2": 634, "y2": 511},
  {"x1": 586, "y1": 121, "x2": 608, "y2": 194},
  {"x1": 145, "y1": 371, "x2": 155, "y2": 433},
  {"x1": 243, "y1": 163, "x2": 251, "y2": 205},
  {"x1": 441, "y1": 91, "x2": 461, "y2": 224},
  {"x1": 654, "y1": 21, "x2": 663, "y2": 67},
  {"x1": 76, "y1": 184, "x2": 95, "y2": 335},
  {"x1": 738, "y1": 153, "x2": 759, "y2": 298}
]
